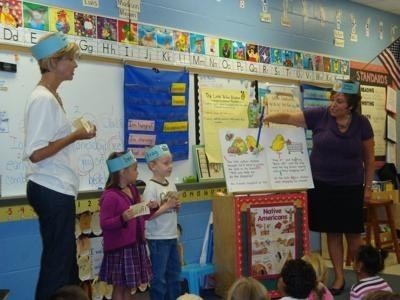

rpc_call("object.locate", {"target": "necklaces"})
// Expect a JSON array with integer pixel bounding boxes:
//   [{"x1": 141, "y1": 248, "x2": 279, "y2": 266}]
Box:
[{"x1": 40, "y1": 78, "x2": 63, "y2": 106}]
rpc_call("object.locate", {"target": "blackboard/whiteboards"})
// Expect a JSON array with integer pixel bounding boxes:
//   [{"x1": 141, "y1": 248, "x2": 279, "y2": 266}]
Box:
[{"x1": 0, "y1": 49, "x2": 195, "y2": 200}]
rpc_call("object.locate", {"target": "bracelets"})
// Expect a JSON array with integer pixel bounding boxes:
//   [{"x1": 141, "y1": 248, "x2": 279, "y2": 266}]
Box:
[{"x1": 363, "y1": 183, "x2": 372, "y2": 189}]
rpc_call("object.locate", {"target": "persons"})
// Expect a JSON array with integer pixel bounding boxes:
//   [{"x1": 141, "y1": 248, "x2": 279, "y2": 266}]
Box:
[
  {"x1": 255, "y1": 81, "x2": 375, "y2": 295},
  {"x1": 99, "y1": 149, "x2": 160, "y2": 300},
  {"x1": 142, "y1": 144, "x2": 181, "y2": 300},
  {"x1": 48, "y1": 244, "x2": 400, "y2": 299},
  {"x1": 23, "y1": 33, "x2": 97, "y2": 299}
]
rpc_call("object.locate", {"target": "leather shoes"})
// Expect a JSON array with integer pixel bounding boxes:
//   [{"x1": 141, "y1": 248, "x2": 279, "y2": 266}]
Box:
[{"x1": 329, "y1": 279, "x2": 345, "y2": 296}]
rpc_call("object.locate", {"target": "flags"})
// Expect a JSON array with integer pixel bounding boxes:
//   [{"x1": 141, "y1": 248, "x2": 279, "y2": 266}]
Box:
[{"x1": 376, "y1": 36, "x2": 400, "y2": 90}]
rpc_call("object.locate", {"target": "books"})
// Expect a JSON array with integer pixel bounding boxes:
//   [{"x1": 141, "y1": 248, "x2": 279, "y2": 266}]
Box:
[
  {"x1": 218, "y1": 125, "x2": 316, "y2": 194},
  {"x1": 192, "y1": 145, "x2": 226, "y2": 183}
]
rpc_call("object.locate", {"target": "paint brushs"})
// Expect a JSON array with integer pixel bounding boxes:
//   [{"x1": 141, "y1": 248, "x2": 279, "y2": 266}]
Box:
[{"x1": 253, "y1": 107, "x2": 265, "y2": 155}]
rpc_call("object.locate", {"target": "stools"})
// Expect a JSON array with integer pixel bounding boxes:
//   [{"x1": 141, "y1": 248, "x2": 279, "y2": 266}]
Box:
[
  {"x1": 345, "y1": 199, "x2": 400, "y2": 266},
  {"x1": 180, "y1": 263, "x2": 216, "y2": 297}
]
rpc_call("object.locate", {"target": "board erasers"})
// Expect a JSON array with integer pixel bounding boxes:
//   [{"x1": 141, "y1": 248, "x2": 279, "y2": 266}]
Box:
[{"x1": 1, "y1": 61, "x2": 18, "y2": 74}]
